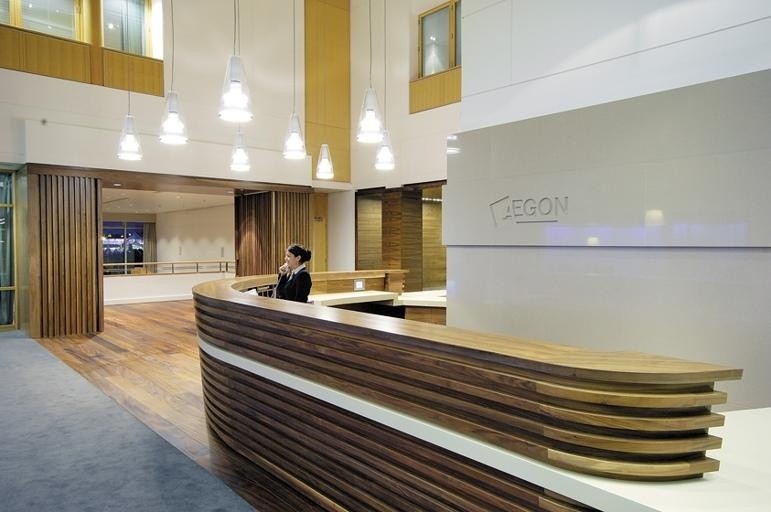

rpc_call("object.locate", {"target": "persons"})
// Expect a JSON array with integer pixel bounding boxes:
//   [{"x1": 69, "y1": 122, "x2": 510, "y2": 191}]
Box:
[{"x1": 275, "y1": 243, "x2": 313, "y2": 303}]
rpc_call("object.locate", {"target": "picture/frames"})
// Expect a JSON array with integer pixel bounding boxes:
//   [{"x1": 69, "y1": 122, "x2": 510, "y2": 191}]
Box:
[{"x1": 352, "y1": 278, "x2": 366, "y2": 291}]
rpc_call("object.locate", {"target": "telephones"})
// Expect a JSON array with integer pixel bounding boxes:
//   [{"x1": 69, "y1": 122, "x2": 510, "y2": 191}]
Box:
[{"x1": 281, "y1": 263, "x2": 288, "y2": 275}]
